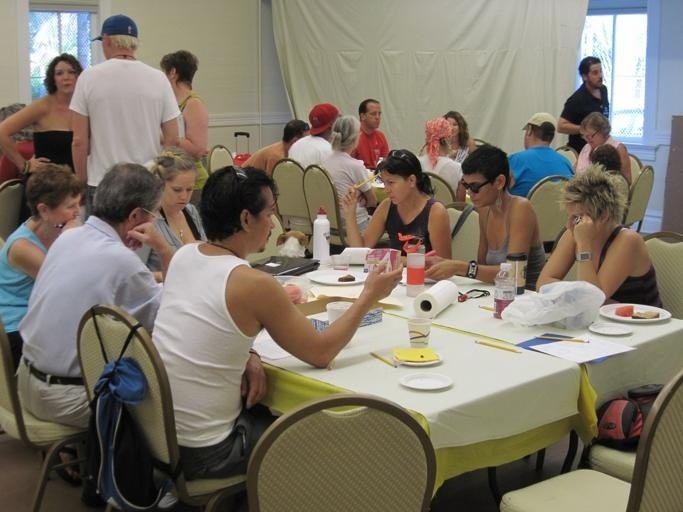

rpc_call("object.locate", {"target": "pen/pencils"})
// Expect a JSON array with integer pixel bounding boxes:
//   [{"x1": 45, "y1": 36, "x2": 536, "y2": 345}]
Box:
[
  {"x1": 369, "y1": 352, "x2": 397, "y2": 369},
  {"x1": 353, "y1": 173, "x2": 381, "y2": 189},
  {"x1": 474, "y1": 339, "x2": 522, "y2": 354},
  {"x1": 534, "y1": 336, "x2": 589, "y2": 343},
  {"x1": 50, "y1": 224, "x2": 64, "y2": 230},
  {"x1": 478, "y1": 304, "x2": 494, "y2": 311}
]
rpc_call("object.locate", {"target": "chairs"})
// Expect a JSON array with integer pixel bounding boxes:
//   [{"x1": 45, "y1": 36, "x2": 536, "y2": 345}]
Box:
[
  {"x1": 500, "y1": 371, "x2": 683, "y2": 512},
  {"x1": 77, "y1": 303, "x2": 246, "y2": 512},
  {"x1": 0, "y1": 319, "x2": 89, "y2": 512},
  {"x1": 589, "y1": 445, "x2": 637, "y2": 483},
  {"x1": 245, "y1": 394, "x2": 437, "y2": 512},
  {"x1": 0, "y1": 178, "x2": 31, "y2": 243}
]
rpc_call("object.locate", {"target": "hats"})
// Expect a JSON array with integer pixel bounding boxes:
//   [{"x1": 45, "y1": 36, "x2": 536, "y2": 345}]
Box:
[
  {"x1": 308, "y1": 103, "x2": 339, "y2": 136},
  {"x1": 92, "y1": 14, "x2": 138, "y2": 41},
  {"x1": 522, "y1": 112, "x2": 558, "y2": 130}
]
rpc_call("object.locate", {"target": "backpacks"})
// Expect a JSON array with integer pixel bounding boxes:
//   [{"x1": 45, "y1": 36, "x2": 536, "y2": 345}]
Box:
[
  {"x1": 82, "y1": 304, "x2": 166, "y2": 512},
  {"x1": 596, "y1": 396, "x2": 644, "y2": 452}
]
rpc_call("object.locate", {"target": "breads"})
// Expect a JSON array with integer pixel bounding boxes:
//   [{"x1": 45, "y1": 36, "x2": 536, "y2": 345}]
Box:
[
  {"x1": 632, "y1": 312, "x2": 663, "y2": 319},
  {"x1": 338, "y1": 274, "x2": 355, "y2": 283}
]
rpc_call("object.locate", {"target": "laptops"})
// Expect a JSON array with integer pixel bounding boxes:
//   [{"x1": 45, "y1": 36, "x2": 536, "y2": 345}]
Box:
[{"x1": 249, "y1": 255, "x2": 322, "y2": 277}]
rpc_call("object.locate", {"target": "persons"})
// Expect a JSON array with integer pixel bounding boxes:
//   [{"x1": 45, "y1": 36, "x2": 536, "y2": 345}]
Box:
[
  {"x1": 0, "y1": 53, "x2": 84, "y2": 226},
  {"x1": 557, "y1": 56, "x2": 610, "y2": 157},
  {"x1": 160, "y1": 51, "x2": 209, "y2": 208},
  {"x1": 0, "y1": 98, "x2": 666, "y2": 480},
  {"x1": 69, "y1": 14, "x2": 181, "y2": 225}
]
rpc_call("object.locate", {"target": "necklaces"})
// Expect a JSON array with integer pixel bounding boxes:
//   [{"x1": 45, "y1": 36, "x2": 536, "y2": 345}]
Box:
[{"x1": 111, "y1": 54, "x2": 137, "y2": 60}]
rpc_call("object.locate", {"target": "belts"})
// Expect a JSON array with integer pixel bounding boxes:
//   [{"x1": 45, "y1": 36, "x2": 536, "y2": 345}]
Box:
[{"x1": 24, "y1": 357, "x2": 84, "y2": 386}]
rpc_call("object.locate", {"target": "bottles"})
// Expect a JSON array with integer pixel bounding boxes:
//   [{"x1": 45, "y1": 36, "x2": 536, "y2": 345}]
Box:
[
  {"x1": 507, "y1": 253, "x2": 527, "y2": 295},
  {"x1": 493, "y1": 263, "x2": 516, "y2": 319},
  {"x1": 406, "y1": 237, "x2": 425, "y2": 298},
  {"x1": 313, "y1": 206, "x2": 330, "y2": 264}
]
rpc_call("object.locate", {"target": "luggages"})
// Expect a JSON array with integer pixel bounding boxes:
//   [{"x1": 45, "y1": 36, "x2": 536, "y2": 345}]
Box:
[{"x1": 233, "y1": 132, "x2": 252, "y2": 166}]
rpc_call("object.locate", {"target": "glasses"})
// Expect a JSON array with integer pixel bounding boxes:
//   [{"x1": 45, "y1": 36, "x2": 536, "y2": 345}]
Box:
[
  {"x1": 461, "y1": 179, "x2": 491, "y2": 194},
  {"x1": 581, "y1": 128, "x2": 601, "y2": 142}
]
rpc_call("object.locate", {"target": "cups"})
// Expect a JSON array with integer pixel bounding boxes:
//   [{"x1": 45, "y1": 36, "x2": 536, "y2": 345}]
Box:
[
  {"x1": 408, "y1": 318, "x2": 431, "y2": 347},
  {"x1": 332, "y1": 255, "x2": 350, "y2": 272},
  {"x1": 326, "y1": 301, "x2": 353, "y2": 349}
]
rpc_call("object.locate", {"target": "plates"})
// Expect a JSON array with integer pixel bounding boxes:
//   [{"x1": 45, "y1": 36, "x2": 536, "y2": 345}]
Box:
[
  {"x1": 399, "y1": 372, "x2": 453, "y2": 390},
  {"x1": 393, "y1": 353, "x2": 442, "y2": 366},
  {"x1": 307, "y1": 270, "x2": 367, "y2": 285},
  {"x1": 589, "y1": 322, "x2": 633, "y2": 335},
  {"x1": 599, "y1": 303, "x2": 672, "y2": 324}
]
